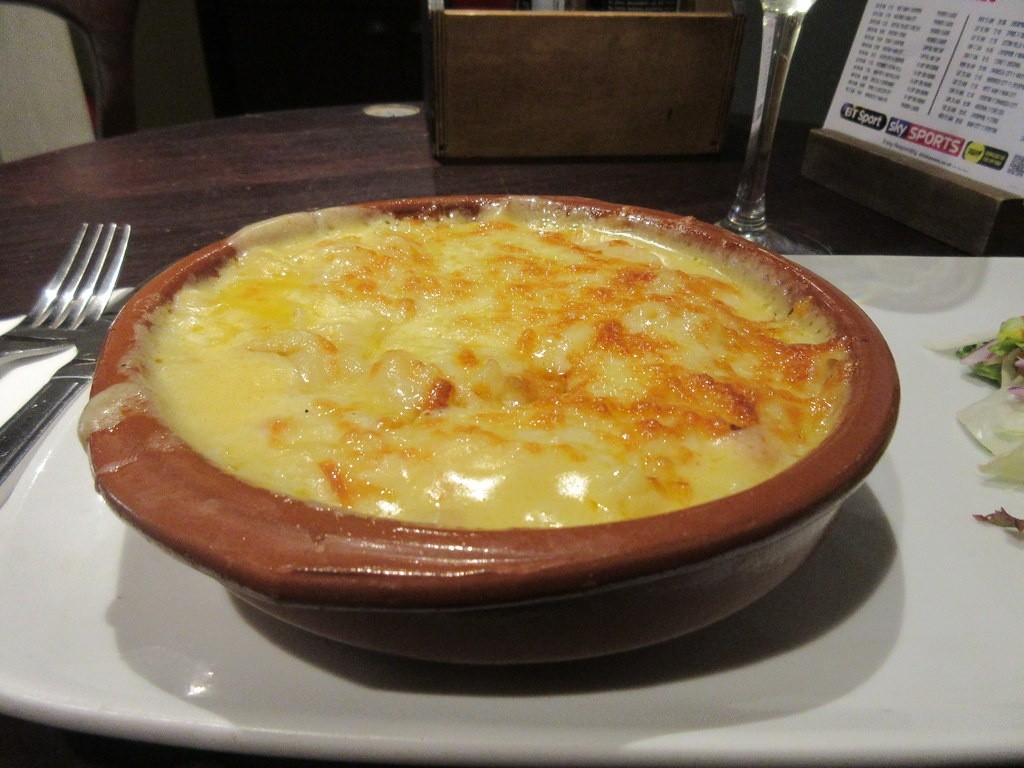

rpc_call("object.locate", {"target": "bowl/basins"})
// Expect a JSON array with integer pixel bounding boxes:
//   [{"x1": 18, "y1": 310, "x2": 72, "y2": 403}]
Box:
[{"x1": 84, "y1": 193, "x2": 901, "y2": 666}]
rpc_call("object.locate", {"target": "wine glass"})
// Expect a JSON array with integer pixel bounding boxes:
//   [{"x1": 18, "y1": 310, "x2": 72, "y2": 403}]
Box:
[{"x1": 699, "y1": 0, "x2": 838, "y2": 256}]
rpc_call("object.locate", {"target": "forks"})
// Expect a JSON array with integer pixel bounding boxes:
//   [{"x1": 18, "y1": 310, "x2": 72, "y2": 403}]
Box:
[{"x1": 0, "y1": 221, "x2": 132, "y2": 374}]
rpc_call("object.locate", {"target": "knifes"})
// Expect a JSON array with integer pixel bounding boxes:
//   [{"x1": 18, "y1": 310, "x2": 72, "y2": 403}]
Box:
[{"x1": 0, "y1": 261, "x2": 179, "y2": 484}]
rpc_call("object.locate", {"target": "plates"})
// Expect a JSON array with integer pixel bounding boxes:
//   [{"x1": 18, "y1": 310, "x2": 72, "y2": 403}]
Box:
[{"x1": 0, "y1": 253, "x2": 1024, "y2": 763}]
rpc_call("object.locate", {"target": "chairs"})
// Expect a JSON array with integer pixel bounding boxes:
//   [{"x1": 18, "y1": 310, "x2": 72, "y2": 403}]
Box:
[{"x1": 0, "y1": 0, "x2": 105, "y2": 164}]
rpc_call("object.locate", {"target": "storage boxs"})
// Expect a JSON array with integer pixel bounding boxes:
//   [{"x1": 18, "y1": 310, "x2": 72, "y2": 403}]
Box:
[{"x1": 421, "y1": 0, "x2": 749, "y2": 166}]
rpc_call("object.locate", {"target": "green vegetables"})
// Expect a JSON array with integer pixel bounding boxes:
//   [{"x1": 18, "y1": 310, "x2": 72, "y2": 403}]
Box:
[{"x1": 923, "y1": 315, "x2": 1024, "y2": 539}]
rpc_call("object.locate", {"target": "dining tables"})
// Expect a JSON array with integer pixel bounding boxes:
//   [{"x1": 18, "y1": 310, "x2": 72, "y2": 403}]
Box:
[{"x1": 0, "y1": 101, "x2": 1024, "y2": 768}]
